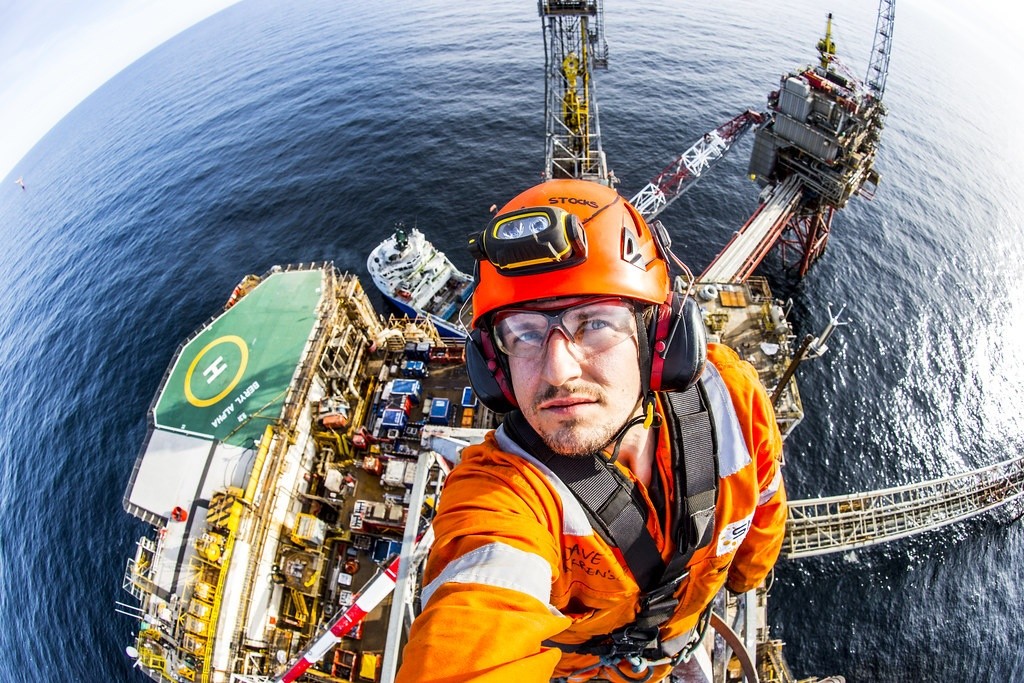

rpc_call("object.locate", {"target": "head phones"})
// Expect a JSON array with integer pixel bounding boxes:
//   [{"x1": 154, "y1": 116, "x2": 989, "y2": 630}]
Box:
[{"x1": 460, "y1": 220, "x2": 706, "y2": 414}]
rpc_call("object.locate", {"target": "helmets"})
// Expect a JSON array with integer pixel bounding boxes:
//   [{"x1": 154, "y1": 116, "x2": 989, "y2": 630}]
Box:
[{"x1": 468, "y1": 178, "x2": 672, "y2": 330}]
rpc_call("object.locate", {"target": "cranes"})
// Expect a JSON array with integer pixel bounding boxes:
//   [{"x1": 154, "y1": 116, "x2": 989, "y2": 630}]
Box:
[{"x1": 615, "y1": 111, "x2": 766, "y2": 221}]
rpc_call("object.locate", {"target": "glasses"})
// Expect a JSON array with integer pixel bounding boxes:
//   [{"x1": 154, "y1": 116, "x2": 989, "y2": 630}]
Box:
[{"x1": 492, "y1": 296, "x2": 636, "y2": 361}]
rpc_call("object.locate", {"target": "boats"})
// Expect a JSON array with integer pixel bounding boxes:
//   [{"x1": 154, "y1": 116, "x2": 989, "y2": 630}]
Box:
[{"x1": 366, "y1": 222, "x2": 476, "y2": 343}]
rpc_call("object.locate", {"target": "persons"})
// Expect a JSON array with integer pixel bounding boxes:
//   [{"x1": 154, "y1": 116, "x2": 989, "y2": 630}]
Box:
[{"x1": 394, "y1": 178, "x2": 789, "y2": 682}]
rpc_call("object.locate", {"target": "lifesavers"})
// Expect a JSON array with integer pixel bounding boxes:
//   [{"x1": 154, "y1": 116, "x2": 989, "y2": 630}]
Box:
[{"x1": 699, "y1": 284, "x2": 718, "y2": 302}]
